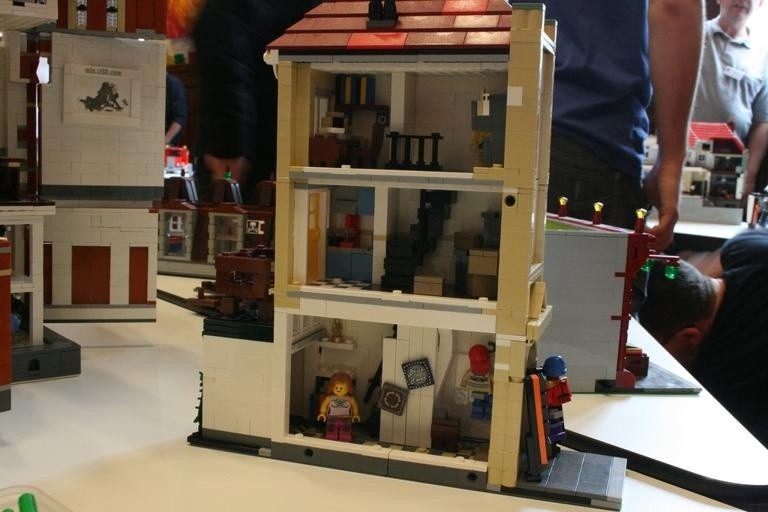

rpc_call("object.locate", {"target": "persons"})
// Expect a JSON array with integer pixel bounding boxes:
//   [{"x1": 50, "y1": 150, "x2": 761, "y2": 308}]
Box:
[
  {"x1": 691, "y1": 0, "x2": 768, "y2": 196},
  {"x1": 461, "y1": 346, "x2": 493, "y2": 421},
  {"x1": 631, "y1": 229, "x2": 767, "y2": 449},
  {"x1": 317, "y1": 372, "x2": 360, "y2": 442},
  {"x1": 542, "y1": 356, "x2": 572, "y2": 442},
  {"x1": 166, "y1": 1, "x2": 323, "y2": 206},
  {"x1": 512, "y1": 0, "x2": 707, "y2": 252}
]
